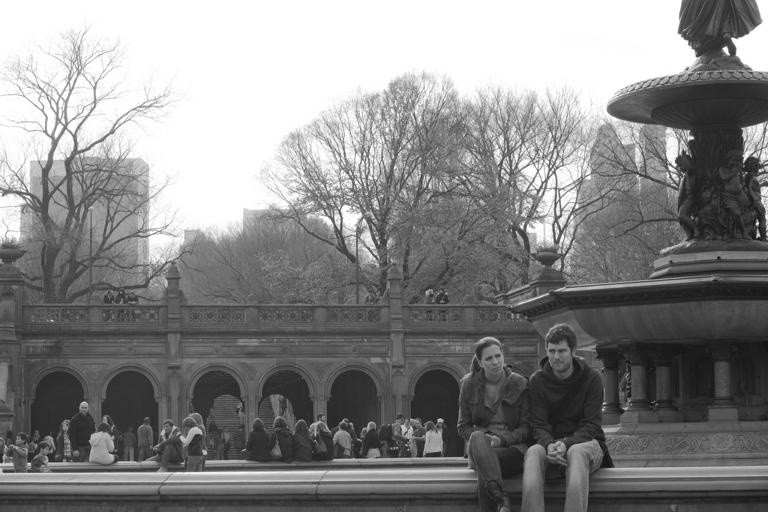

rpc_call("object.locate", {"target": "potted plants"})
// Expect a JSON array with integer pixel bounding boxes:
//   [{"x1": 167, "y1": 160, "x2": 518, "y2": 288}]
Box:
[
  {"x1": 532, "y1": 242, "x2": 563, "y2": 267},
  {"x1": 0, "y1": 237, "x2": 26, "y2": 263}
]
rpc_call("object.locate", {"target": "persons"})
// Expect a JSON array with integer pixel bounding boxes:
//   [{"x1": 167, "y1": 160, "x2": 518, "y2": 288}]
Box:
[
  {"x1": 127, "y1": 290, "x2": 139, "y2": 322},
  {"x1": 362, "y1": 289, "x2": 380, "y2": 321},
  {"x1": 436, "y1": 287, "x2": 449, "y2": 321},
  {"x1": 145, "y1": 413, "x2": 208, "y2": 473},
  {"x1": 115, "y1": 287, "x2": 127, "y2": 321},
  {"x1": 103, "y1": 289, "x2": 114, "y2": 321},
  {"x1": 624, "y1": 360, "x2": 632, "y2": 398},
  {"x1": 676, "y1": 146, "x2": 768, "y2": 244},
  {"x1": 209, "y1": 413, "x2": 313, "y2": 464},
  {"x1": 123, "y1": 417, "x2": 153, "y2": 462},
  {"x1": 1, "y1": 429, "x2": 56, "y2": 473},
  {"x1": 309, "y1": 412, "x2": 449, "y2": 461},
  {"x1": 56, "y1": 401, "x2": 123, "y2": 464},
  {"x1": 519, "y1": 322, "x2": 608, "y2": 512},
  {"x1": 456, "y1": 335, "x2": 536, "y2": 511},
  {"x1": 677, "y1": 0, "x2": 763, "y2": 58},
  {"x1": 425, "y1": 288, "x2": 434, "y2": 321},
  {"x1": 236, "y1": 401, "x2": 242, "y2": 414}
]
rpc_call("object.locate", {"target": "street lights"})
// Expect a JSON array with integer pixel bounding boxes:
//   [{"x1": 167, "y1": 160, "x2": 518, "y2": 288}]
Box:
[
  {"x1": 356, "y1": 213, "x2": 372, "y2": 305},
  {"x1": 88, "y1": 206, "x2": 94, "y2": 306}
]
